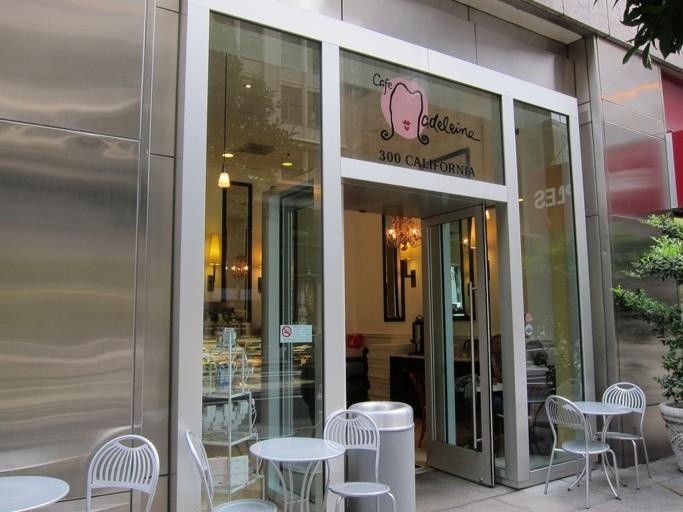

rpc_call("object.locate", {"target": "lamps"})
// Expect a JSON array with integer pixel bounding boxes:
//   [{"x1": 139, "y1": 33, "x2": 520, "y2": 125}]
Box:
[
  {"x1": 226, "y1": 252, "x2": 250, "y2": 281},
  {"x1": 208, "y1": 233, "x2": 222, "y2": 293},
  {"x1": 218, "y1": 51, "x2": 234, "y2": 189},
  {"x1": 384, "y1": 217, "x2": 424, "y2": 290}
]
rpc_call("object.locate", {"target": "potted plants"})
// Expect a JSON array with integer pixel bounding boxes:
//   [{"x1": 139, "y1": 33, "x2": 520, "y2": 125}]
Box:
[{"x1": 609, "y1": 212, "x2": 683, "y2": 473}]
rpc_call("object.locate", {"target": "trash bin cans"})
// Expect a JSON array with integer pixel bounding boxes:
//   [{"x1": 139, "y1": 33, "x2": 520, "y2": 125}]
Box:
[{"x1": 347, "y1": 401, "x2": 416, "y2": 512}]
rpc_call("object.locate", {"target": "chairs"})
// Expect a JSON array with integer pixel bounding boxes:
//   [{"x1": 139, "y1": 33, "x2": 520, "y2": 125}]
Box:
[{"x1": 87, "y1": 432, "x2": 163, "y2": 512}]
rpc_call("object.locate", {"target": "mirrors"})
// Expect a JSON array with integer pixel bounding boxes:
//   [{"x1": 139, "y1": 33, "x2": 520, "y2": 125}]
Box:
[
  {"x1": 221, "y1": 181, "x2": 255, "y2": 324},
  {"x1": 415, "y1": 146, "x2": 476, "y2": 321},
  {"x1": 381, "y1": 212, "x2": 407, "y2": 322}
]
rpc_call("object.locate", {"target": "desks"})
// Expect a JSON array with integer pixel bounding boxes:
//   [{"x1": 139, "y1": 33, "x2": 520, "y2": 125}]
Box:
[{"x1": 1, "y1": 474, "x2": 71, "y2": 512}]
